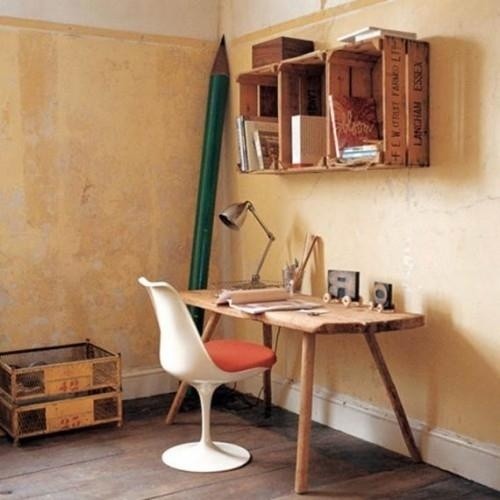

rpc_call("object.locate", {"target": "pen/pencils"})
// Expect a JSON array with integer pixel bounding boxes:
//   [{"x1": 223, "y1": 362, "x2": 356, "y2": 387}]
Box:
[
  {"x1": 286, "y1": 264, "x2": 290, "y2": 272},
  {"x1": 180, "y1": 33, "x2": 229, "y2": 411},
  {"x1": 295, "y1": 258, "x2": 298, "y2": 268}
]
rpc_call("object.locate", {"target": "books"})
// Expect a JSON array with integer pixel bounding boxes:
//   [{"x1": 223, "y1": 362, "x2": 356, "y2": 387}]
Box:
[
  {"x1": 234, "y1": 94, "x2": 383, "y2": 172},
  {"x1": 337, "y1": 27, "x2": 418, "y2": 42},
  {"x1": 214, "y1": 286, "x2": 312, "y2": 315}
]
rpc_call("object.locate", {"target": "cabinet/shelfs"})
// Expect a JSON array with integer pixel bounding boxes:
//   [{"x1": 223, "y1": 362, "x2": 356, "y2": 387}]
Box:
[{"x1": 235, "y1": 36, "x2": 430, "y2": 175}]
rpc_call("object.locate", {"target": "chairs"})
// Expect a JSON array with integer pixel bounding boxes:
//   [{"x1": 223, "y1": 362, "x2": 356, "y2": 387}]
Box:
[{"x1": 137, "y1": 276, "x2": 278, "y2": 473}]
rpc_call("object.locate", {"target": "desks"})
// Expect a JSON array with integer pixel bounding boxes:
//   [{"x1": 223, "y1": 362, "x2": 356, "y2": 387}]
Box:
[{"x1": 165, "y1": 288, "x2": 426, "y2": 494}]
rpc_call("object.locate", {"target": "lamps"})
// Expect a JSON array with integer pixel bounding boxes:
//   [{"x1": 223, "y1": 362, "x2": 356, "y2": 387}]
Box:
[{"x1": 219, "y1": 200, "x2": 276, "y2": 288}]
